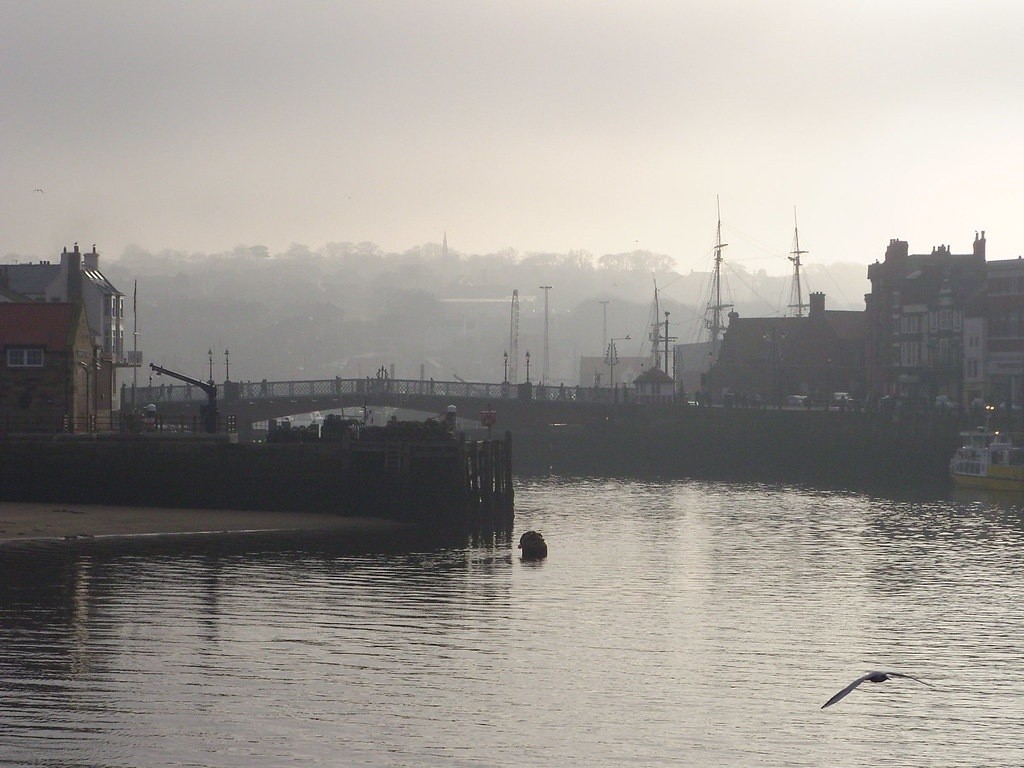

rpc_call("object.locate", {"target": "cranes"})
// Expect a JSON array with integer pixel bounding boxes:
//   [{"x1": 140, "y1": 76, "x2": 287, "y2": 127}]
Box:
[{"x1": 150, "y1": 362, "x2": 222, "y2": 434}]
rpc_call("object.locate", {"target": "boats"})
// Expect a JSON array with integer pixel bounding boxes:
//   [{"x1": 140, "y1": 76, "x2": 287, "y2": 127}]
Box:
[{"x1": 947, "y1": 404, "x2": 1024, "y2": 491}]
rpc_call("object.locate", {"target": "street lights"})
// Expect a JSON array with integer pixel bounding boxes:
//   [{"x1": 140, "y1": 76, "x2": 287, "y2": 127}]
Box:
[
  {"x1": 504, "y1": 352, "x2": 509, "y2": 382},
  {"x1": 526, "y1": 351, "x2": 531, "y2": 384}
]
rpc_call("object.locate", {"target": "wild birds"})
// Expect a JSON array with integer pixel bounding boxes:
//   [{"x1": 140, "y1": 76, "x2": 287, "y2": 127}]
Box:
[{"x1": 820, "y1": 670, "x2": 936, "y2": 712}]
